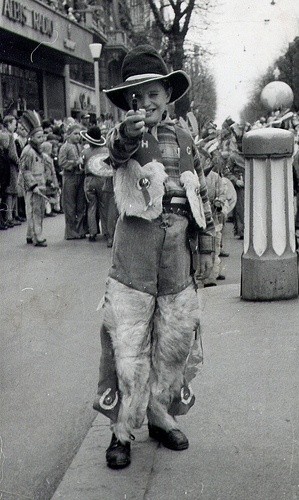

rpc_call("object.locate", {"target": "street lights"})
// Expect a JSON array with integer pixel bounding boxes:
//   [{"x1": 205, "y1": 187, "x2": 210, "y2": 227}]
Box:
[{"x1": 90, "y1": 42, "x2": 103, "y2": 125}]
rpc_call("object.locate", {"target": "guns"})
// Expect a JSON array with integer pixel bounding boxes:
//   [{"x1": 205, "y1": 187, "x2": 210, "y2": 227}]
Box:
[{"x1": 131, "y1": 94, "x2": 144, "y2": 140}]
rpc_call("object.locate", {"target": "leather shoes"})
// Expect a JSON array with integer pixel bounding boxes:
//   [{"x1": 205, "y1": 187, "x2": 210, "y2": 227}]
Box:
[
  {"x1": 105, "y1": 432, "x2": 135, "y2": 469},
  {"x1": 148, "y1": 423, "x2": 189, "y2": 450}
]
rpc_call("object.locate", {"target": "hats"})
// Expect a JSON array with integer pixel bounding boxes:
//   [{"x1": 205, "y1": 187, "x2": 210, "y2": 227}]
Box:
[
  {"x1": 197, "y1": 138, "x2": 219, "y2": 158},
  {"x1": 80, "y1": 126, "x2": 107, "y2": 147},
  {"x1": 102, "y1": 44, "x2": 192, "y2": 112},
  {"x1": 20, "y1": 109, "x2": 44, "y2": 140},
  {"x1": 66, "y1": 122, "x2": 82, "y2": 132}
]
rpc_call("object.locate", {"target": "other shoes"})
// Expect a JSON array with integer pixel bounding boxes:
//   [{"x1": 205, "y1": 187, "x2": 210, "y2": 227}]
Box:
[
  {"x1": 52, "y1": 208, "x2": 64, "y2": 214},
  {"x1": 2, "y1": 221, "x2": 13, "y2": 228},
  {"x1": 240, "y1": 235, "x2": 244, "y2": 240},
  {"x1": 31, "y1": 236, "x2": 48, "y2": 247},
  {"x1": 219, "y1": 249, "x2": 230, "y2": 257},
  {"x1": 216, "y1": 274, "x2": 225, "y2": 280},
  {"x1": 26, "y1": 236, "x2": 34, "y2": 244},
  {"x1": 9, "y1": 218, "x2": 22, "y2": 225},
  {"x1": 89, "y1": 235, "x2": 99, "y2": 242},
  {"x1": 45, "y1": 212, "x2": 57, "y2": 217},
  {"x1": 14, "y1": 216, "x2": 26, "y2": 222},
  {"x1": 0, "y1": 221, "x2": 8, "y2": 230},
  {"x1": 107, "y1": 237, "x2": 113, "y2": 247},
  {"x1": 74, "y1": 234, "x2": 86, "y2": 239}
]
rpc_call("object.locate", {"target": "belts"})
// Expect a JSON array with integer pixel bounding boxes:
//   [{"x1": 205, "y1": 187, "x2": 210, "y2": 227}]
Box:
[{"x1": 163, "y1": 205, "x2": 188, "y2": 218}]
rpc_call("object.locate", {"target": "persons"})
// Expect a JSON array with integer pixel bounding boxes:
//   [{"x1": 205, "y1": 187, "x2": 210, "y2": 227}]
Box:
[
  {"x1": 0, "y1": 107, "x2": 299, "y2": 287},
  {"x1": 97, "y1": 44, "x2": 215, "y2": 468}
]
